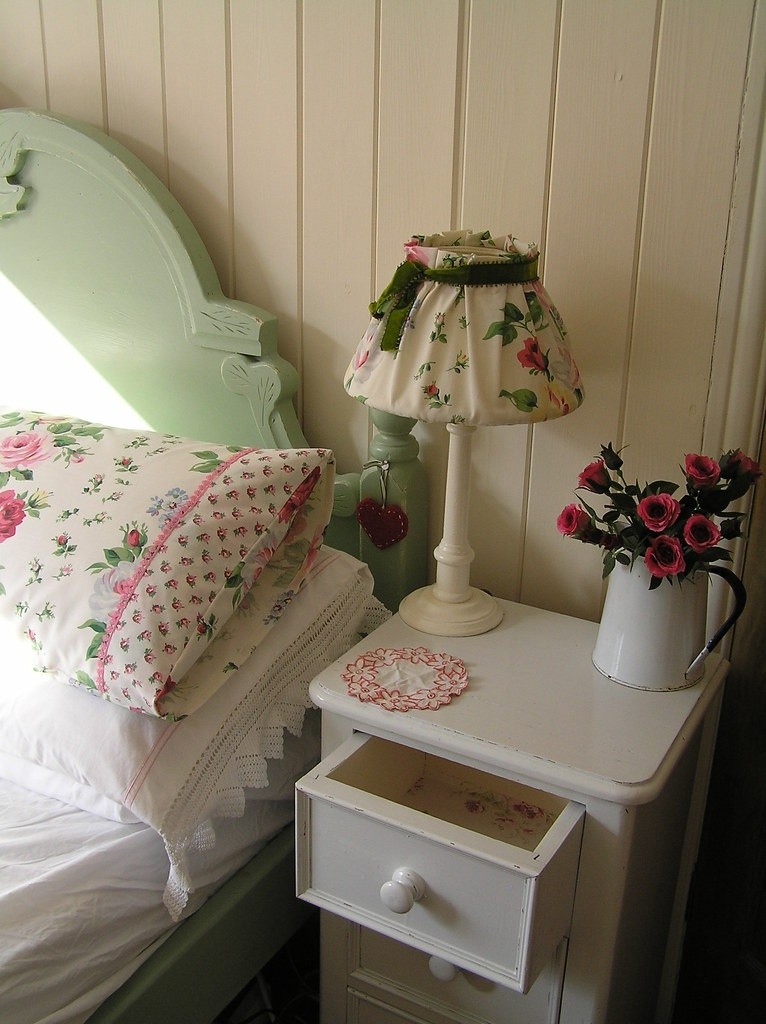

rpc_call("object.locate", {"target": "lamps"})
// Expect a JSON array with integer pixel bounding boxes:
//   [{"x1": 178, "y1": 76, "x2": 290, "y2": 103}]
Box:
[{"x1": 342, "y1": 228, "x2": 587, "y2": 638}]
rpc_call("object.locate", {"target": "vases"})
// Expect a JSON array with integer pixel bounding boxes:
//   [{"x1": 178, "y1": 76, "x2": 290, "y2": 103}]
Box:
[{"x1": 590, "y1": 544, "x2": 748, "y2": 696}]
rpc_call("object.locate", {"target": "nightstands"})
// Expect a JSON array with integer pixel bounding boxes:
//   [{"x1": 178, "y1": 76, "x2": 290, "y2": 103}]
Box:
[{"x1": 292, "y1": 594, "x2": 735, "y2": 1024}]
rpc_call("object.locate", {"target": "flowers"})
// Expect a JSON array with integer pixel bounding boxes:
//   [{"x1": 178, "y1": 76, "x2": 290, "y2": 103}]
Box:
[{"x1": 555, "y1": 441, "x2": 766, "y2": 595}]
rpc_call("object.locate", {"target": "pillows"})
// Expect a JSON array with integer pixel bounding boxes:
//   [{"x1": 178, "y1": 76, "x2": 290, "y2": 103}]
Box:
[
  {"x1": 0, "y1": 407, "x2": 338, "y2": 725},
  {"x1": 0, "y1": 543, "x2": 397, "y2": 921}
]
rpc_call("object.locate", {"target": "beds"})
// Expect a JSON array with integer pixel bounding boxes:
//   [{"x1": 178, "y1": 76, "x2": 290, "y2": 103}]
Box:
[{"x1": 0, "y1": 104, "x2": 435, "y2": 1024}]
